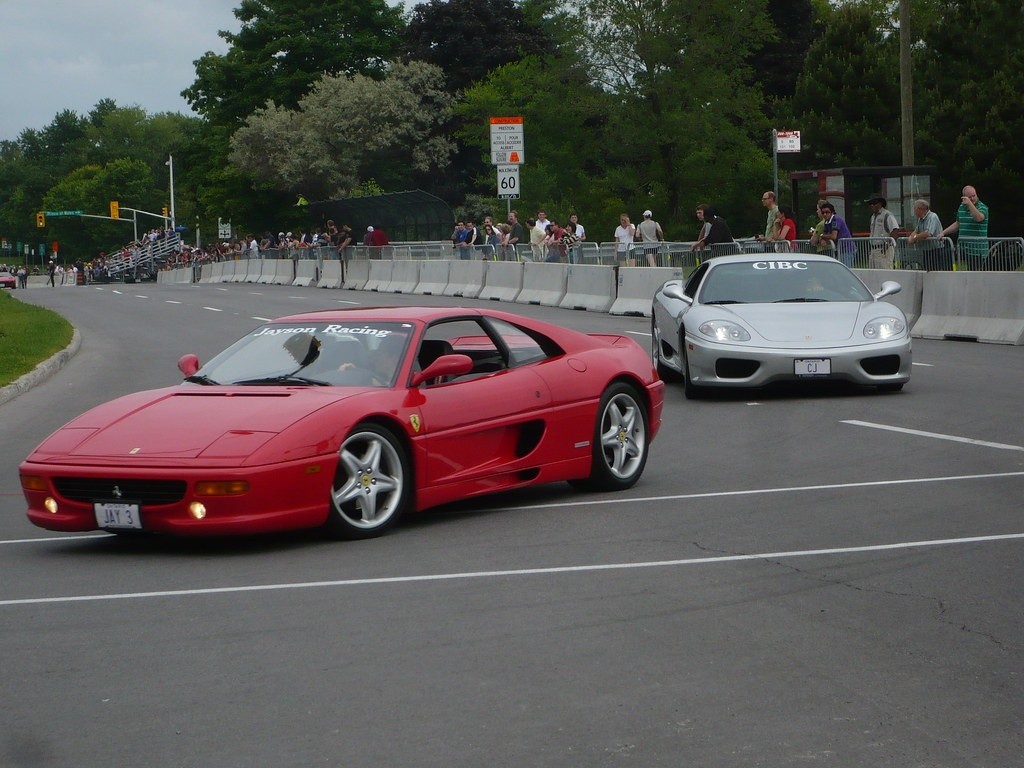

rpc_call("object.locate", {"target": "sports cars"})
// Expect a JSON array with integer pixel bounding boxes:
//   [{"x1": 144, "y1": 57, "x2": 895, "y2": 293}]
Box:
[
  {"x1": 17, "y1": 306, "x2": 664, "y2": 540},
  {"x1": 0, "y1": 272, "x2": 16, "y2": 289},
  {"x1": 651, "y1": 253, "x2": 912, "y2": 400}
]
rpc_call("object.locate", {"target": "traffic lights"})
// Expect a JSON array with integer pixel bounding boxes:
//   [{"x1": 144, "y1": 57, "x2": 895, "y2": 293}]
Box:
[
  {"x1": 37, "y1": 214, "x2": 45, "y2": 227},
  {"x1": 162, "y1": 208, "x2": 167, "y2": 217}
]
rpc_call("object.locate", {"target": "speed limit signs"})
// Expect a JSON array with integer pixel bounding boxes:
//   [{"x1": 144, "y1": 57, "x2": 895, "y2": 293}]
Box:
[{"x1": 497, "y1": 165, "x2": 520, "y2": 199}]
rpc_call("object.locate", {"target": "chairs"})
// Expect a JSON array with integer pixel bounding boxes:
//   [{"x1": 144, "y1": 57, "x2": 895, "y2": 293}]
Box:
[{"x1": 418, "y1": 339, "x2": 455, "y2": 384}]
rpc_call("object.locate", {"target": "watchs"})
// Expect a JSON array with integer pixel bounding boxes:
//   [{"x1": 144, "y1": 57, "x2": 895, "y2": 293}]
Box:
[{"x1": 914, "y1": 235, "x2": 918, "y2": 240}]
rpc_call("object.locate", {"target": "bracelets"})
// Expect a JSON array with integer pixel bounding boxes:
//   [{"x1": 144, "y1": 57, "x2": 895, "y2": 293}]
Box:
[{"x1": 886, "y1": 241, "x2": 888, "y2": 244}]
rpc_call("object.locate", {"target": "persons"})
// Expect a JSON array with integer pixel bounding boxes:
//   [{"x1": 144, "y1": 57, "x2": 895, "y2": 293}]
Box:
[
  {"x1": 0, "y1": 263, "x2": 40, "y2": 289},
  {"x1": 525, "y1": 209, "x2": 586, "y2": 264},
  {"x1": 484, "y1": 210, "x2": 523, "y2": 261},
  {"x1": 339, "y1": 335, "x2": 426, "y2": 386},
  {"x1": 614, "y1": 213, "x2": 636, "y2": 267},
  {"x1": 937, "y1": 185, "x2": 989, "y2": 271},
  {"x1": 803, "y1": 274, "x2": 824, "y2": 292},
  {"x1": 756, "y1": 191, "x2": 800, "y2": 253},
  {"x1": 635, "y1": 210, "x2": 664, "y2": 267},
  {"x1": 295, "y1": 193, "x2": 309, "y2": 206},
  {"x1": 908, "y1": 199, "x2": 950, "y2": 271},
  {"x1": 688, "y1": 204, "x2": 739, "y2": 262},
  {"x1": 864, "y1": 193, "x2": 900, "y2": 269},
  {"x1": 167, "y1": 219, "x2": 357, "y2": 271},
  {"x1": 810, "y1": 200, "x2": 856, "y2": 268},
  {"x1": 450, "y1": 221, "x2": 483, "y2": 260},
  {"x1": 47, "y1": 225, "x2": 176, "y2": 287},
  {"x1": 363, "y1": 222, "x2": 388, "y2": 260}
]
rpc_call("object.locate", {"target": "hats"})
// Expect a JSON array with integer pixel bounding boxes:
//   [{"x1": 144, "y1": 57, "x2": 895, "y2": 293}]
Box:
[
  {"x1": 643, "y1": 210, "x2": 652, "y2": 217},
  {"x1": 864, "y1": 193, "x2": 887, "y2": 208},
  {"x1": 285, "y1": 232, "x2": 292, "y2": 237},
  {"x1": 367, "y1": 226, "x2": 374, "y2": 231},
  {"x1": 49, "y1": 261, "x2": 53, "y2": 263},
  {"x1": 278, "y1": 232, "x2": 284, "y2": 236}
]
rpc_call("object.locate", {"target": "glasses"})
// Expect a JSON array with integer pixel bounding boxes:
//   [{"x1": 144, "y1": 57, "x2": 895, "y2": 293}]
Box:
[
  {"x1": 484, "y1": 222, "x2": 489, "y2": 223},
  {"x1": 549, "y1": 225, "x2": 554, "y2": 226},
  {"x1": 486, "y1": 229, "x2": 490, "y2": 231},
  {"x1": 868, "y1": 201, "x2": 879, "y2": 205},
  {"x1": 762, "y1": 198, "x2": 770, "y2": 201},
  {"x1": 821, "y1": 211, "x2": 831, "y2": 214}
]
